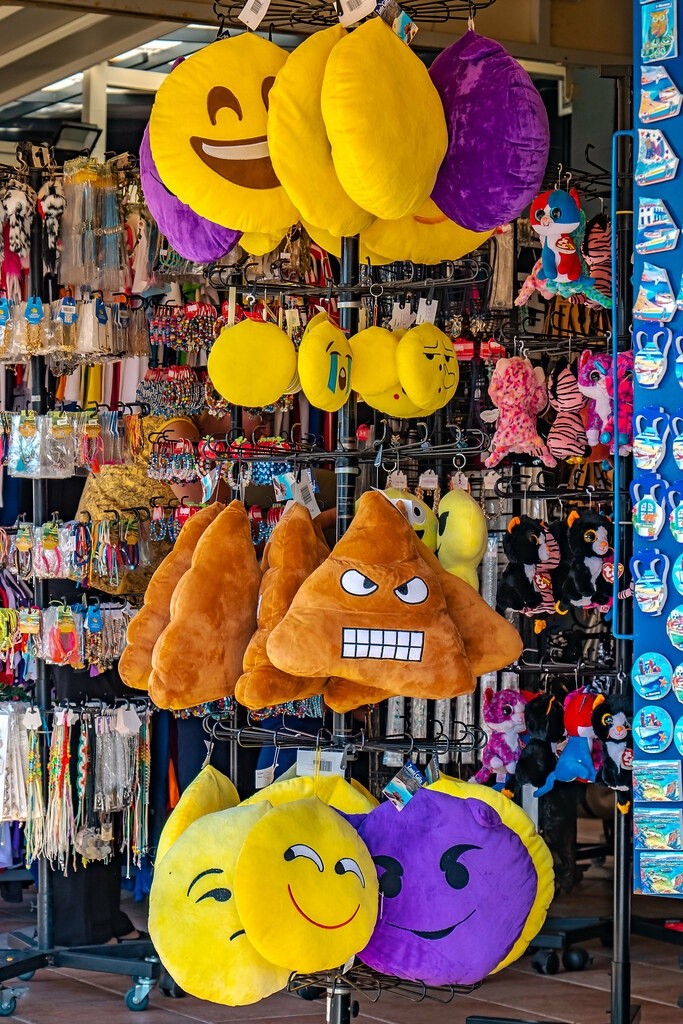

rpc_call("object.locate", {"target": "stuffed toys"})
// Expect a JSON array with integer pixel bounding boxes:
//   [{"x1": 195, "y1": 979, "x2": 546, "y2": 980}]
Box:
[
  {"x1": 0, "y1": 177, "x2": 69, "y2": 308},
  {"x1": 115, "y1": 20, "x2": 637, "y2": 1010}
]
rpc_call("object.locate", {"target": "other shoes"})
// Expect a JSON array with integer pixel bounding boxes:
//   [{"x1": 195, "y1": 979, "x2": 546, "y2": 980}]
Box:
[{"x1": 118, "y1": 930, "x2": 151, "y2": 944}]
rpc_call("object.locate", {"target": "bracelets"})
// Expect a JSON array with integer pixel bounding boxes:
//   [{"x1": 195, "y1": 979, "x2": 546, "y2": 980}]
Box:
[
  {"x1": 0, "y1": 300, "x2": 328, "y2": 877},
  {"x1": 72, "y1": 186, "x2": 122, "y2": 272}
]
